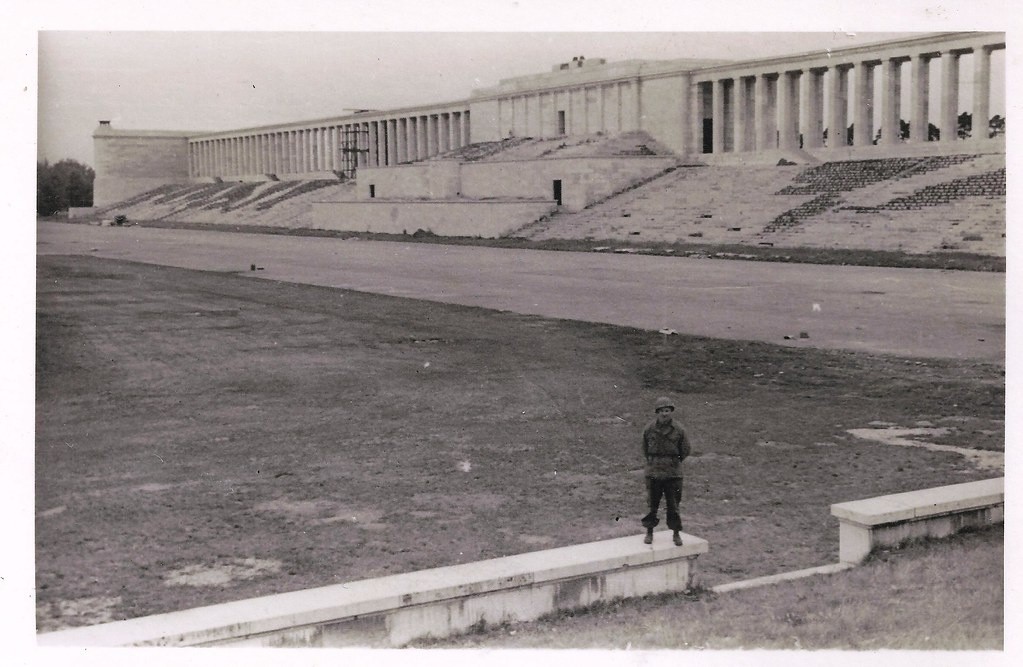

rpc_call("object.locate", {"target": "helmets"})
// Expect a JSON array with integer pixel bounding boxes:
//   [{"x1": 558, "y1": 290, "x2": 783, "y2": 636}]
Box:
[{"x1": 655, "y1": 396, "x2": 675, "y2": 414}]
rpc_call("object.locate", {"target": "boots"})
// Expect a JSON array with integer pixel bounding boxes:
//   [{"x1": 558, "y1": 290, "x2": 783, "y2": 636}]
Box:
[
  {"x1": 645, "y1": 527, "x2": 653, "y2": 544},
  {"x1": 673, "y1": 530, "x2": 682, "y2": 546}
]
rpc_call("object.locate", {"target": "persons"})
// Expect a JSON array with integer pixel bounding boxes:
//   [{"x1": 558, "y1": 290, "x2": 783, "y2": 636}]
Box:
[{"x1": 642, "y1": 397, "x2": 690, "y2": 546}]
